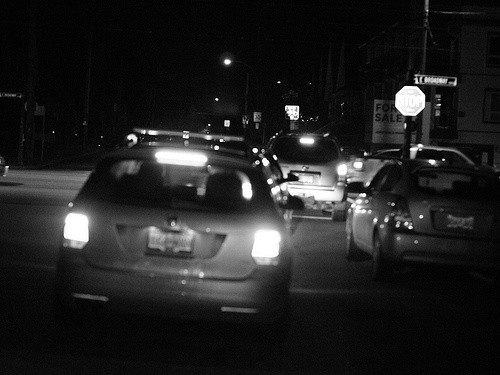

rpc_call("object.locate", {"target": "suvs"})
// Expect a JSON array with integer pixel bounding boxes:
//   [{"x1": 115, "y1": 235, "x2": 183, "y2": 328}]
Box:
[{"x1": 262, "y1": 131, "x2": 347, "y2": 221}]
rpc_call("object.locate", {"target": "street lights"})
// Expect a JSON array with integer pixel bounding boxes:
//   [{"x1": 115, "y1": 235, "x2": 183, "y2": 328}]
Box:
[
  {"x1": 224, "y1": 59, "x2": 255, "y2": 129},
  {"x1": 284, "y1": 105, "x2": 302, "y2": 132}
]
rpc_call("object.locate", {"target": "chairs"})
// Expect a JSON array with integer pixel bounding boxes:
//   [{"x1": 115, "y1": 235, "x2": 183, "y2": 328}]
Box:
[
  {"x1": 452, "y1": 180, "x2": 477, "y2": 198},
  {"x1": 121, "y1": 169, "x2": 164, "y2": 201},
  {"x1": 202, "y1": 171, "x2": 242, "y2": 209}
]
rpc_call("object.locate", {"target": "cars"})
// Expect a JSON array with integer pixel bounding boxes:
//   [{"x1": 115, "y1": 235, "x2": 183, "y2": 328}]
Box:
[
  {"x1": 60, "y1": 129, "x2": 305, "y2": 321},
  {"x1": 350, "y1": 146, "x2": 482, "y2": 193},
  {"x1": 343, "y1": 160, "x2": 500, "y2": 279}
]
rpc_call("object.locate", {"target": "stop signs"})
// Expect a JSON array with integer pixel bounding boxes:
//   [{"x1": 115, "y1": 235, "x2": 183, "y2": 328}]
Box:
[{"x1": 394, "y1": 85, "x2": 426, "y2": 117}]
